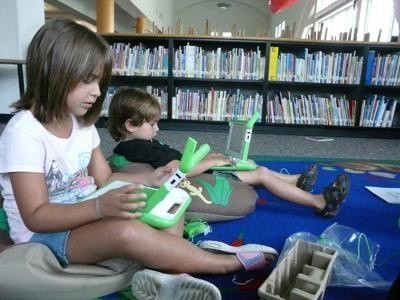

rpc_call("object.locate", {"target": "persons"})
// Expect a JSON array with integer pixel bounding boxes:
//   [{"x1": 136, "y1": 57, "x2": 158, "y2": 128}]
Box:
[
  {"x1": 107, "y1": 87, "x2": 349, "y2": 218},
  {"x1": 0, "y1": 16, "x2": 279, "y2": 300}
]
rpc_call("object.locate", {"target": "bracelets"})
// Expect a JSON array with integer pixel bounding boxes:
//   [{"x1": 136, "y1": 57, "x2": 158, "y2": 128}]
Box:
[{"x1": 96, "y1": 196, "x2": 105, "y2": 220}]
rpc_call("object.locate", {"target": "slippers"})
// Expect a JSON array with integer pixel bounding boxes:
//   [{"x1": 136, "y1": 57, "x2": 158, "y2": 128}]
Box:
[
  {"x1": 199, "y1": 240, "x2": 279, "y2": 272},
  {"x1": 131, "y1": 269, "x2": 222, "y2": 300}
]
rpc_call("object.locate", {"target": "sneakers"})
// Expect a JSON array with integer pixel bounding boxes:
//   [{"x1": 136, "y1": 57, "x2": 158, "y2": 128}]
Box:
[
  {"x1": 316, "y1": 174, "x2": 350, "y2": 219},
  {"x1": 296, "y1": 164, "x2": 317, "y2": 192}
]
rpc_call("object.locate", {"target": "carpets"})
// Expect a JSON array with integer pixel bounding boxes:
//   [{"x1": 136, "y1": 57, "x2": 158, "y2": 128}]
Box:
[{"x1": 196, "y1": 153, "x2": 399, "y2": 299}]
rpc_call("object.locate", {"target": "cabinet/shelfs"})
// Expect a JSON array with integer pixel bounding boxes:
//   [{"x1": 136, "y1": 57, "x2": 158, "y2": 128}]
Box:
[{"x1": 95, "y1": 34, "x2": 399, "y2": 139}]
